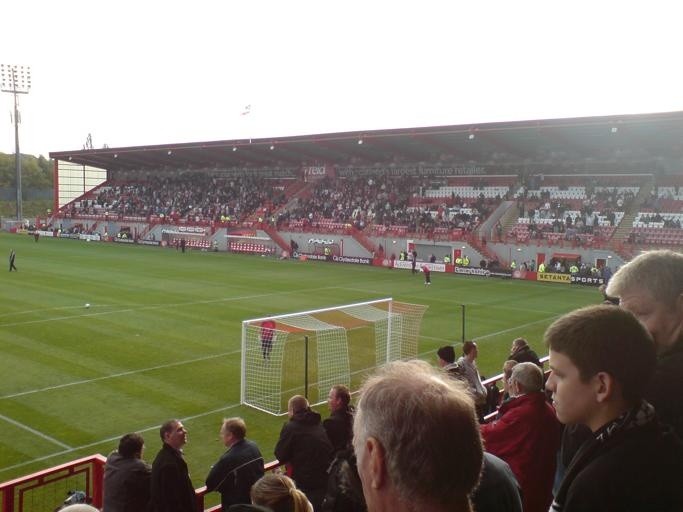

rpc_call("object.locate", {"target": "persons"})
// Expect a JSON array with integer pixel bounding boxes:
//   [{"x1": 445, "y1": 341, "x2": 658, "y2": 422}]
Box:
[
  {"x1": 468, "y1": 452, "x2": 524, "y2": 511},
  {"x1": 322, "y1": 408, "x2": 367, "y2": 511},
  {"x1": 58, "y1": 501, "x2": 100, "y2": 511},
  {"x1": 435, "y1": 335, "x2": 548, "y2": 422},
  {"x1": 258, "y1": 312, "x2": 277, "y2": 361},
  {"x1": 19, "y1": 172, "x2": 683, "y2": 288},
  {"x1": 150, "y1": 419, "x2": 197, "y2": 511},
  {"x1": 205, "y1": 416, "x2": 264, "y2": 510},
  {"x1": 274, "y1": 395, "x2": 338, "y2": 510},
  {"x1": 250, "y1": 473, "x2": 314, "y2": 512},
  {"x1": 479, "y1": 361, "x2": 564, "y2": 512},
  {"x1": 323, "y1": 384, "x2": 355, "y2": 451},
  {"x1": 604, "y1": 248, "x2": 683, "y2": 429},
  {"x1": 8, "y1": 248, "x2": 17, "y2": 271},
  {"x1": 350, "y1": 358, "x2": 484, "y2": 511},
  {"x1": 103, "y1": 432, "x2": 152, "y2": 511},
  {"x1": 541, "y1": 303, "x2": 683, "y2": 512}
]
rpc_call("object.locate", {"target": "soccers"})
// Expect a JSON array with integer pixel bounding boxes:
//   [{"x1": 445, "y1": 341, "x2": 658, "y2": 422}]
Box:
[{"x1": 85, "y1": 303, "x2": 90, "y2": 308}]
